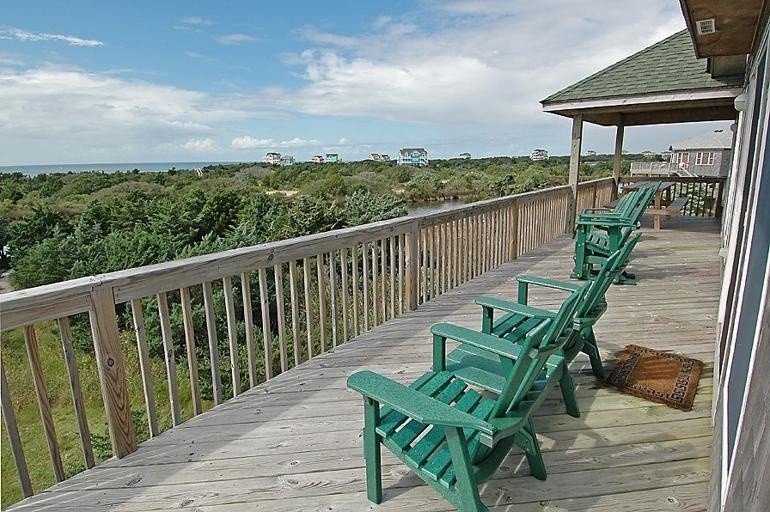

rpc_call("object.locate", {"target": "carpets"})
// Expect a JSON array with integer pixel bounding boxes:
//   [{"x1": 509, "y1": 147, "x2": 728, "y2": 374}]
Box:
[{"x1": 597, "y1": 344, "x2": 706, "y2": 410}]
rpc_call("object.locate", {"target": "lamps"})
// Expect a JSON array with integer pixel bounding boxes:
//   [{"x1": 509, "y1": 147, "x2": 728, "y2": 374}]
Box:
[{"x1": 734, "y1": 92, "x2": 748, "y2": 112}]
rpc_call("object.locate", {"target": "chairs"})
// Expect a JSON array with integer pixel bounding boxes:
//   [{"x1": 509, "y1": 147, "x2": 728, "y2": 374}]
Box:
[
  {"x1": 476, "y1": 250, "x2": 622, "y2": 415},
  {"x1": 568, "y1": 181, "x2": 660, "y2": 287},
  {"x1": 431, "y1": 291, "x2": 580, "y2": 476},
  {"x1": 345, "y1": 316, "x2": 554, "y2": 512}
]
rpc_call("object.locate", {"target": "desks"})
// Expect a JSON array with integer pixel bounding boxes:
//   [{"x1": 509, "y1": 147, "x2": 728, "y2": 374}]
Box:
[{"x1": 620, "y1": 181, "x2": 676, "y2": 232}]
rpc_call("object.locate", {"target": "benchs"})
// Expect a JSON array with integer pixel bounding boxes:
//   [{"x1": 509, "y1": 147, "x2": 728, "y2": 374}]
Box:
[{"x1": 666, "y1": 196, "x2": 689, "y2": 211}]
